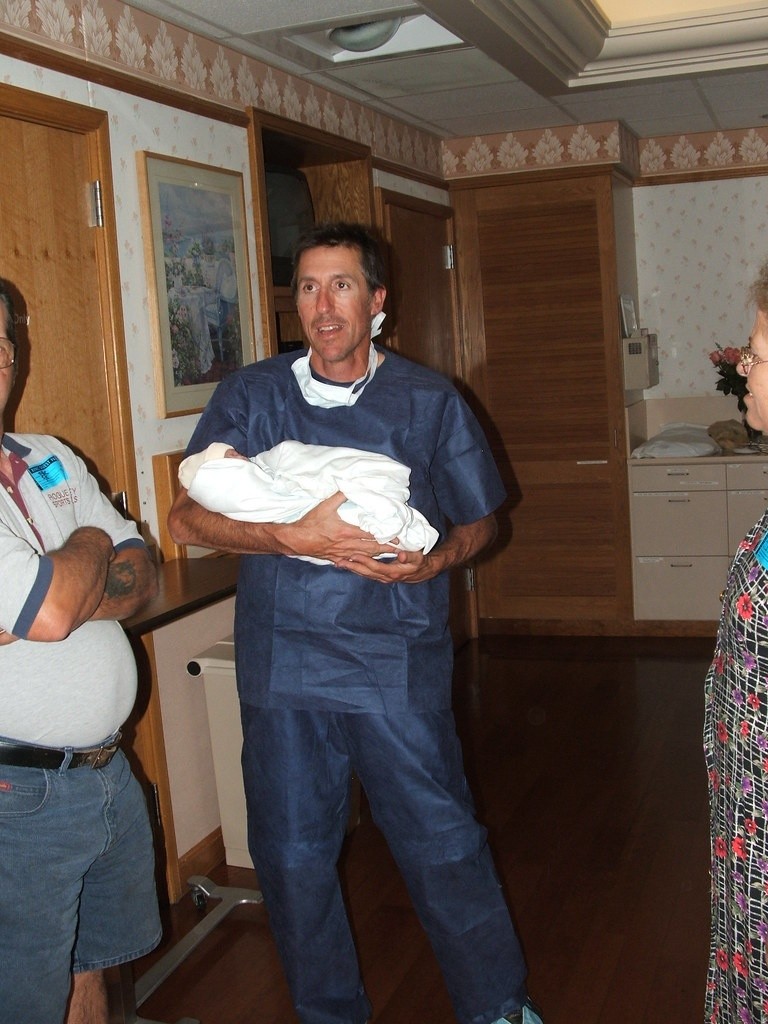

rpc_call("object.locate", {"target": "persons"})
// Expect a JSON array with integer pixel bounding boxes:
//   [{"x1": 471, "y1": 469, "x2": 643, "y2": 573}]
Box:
[
  {"x1": 167, "y1": 222, "x2": 543, "y2": 1024},
  {"x1": 177, "y1": 439, "x2": 440, "y2": 566},
  {"x1": 0, "y1": 293, "x2": 162, "y2": 1024},
  {"x1": 701, "y1": 264, "x2": 768, "y2": 1024}
]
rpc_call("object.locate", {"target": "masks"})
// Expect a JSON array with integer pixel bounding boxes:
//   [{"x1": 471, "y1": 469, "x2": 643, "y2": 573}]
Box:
[{"x1": 290, "y1": 311, "x2": 387, "y2": 409}]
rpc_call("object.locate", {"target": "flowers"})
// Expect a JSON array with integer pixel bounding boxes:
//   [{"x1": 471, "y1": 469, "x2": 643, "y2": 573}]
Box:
[{"x1": 709, "y1": 341, "x2": 760, "y2": 438}]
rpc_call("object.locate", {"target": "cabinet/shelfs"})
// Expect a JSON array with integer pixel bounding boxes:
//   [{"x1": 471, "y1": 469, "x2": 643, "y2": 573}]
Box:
[
  {"x1": 628, "y1": 463, "x2": 768, "y2": 625},
  {"x1": 246, "y1": 110, "x2": 387, "y2": 357},
  {"x1": 188, "y1": 633, "x2": 361, "y2": 870},
  {"x1": 446, "y1": 166, "x2": 644, "y2": 645}
]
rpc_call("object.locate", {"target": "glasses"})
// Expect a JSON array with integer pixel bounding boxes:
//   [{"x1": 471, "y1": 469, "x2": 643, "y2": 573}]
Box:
[
  {"x1": 740, "y1": 346, "x2": 767, "y2": 374},
  {"x1": 0, "y1": 337, "x2": 17, "y2": 369}
]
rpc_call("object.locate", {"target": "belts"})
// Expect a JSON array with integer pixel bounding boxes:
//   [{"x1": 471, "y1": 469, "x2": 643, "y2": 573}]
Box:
[{"x1": 0, "y1": 733, "x2": 125, "y2": 769}]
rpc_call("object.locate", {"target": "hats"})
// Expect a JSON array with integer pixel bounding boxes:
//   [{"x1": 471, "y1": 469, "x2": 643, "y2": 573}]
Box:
[{"x1": 177, "y1": 442, "x2": 234, "y2": 489}]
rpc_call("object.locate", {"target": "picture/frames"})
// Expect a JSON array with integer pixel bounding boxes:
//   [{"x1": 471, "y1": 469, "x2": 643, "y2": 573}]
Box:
[
  {"x1": 138, "y1": 149, "x2": 258, "y2": 420},
  {"x1": 153, "y1": 450, "x2": 230, "y2": 562}
]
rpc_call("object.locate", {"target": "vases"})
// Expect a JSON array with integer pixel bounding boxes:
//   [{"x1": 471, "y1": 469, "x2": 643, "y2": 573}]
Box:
[{"x1": 742, "y1": 407, "x2": 762, "y2": 445}]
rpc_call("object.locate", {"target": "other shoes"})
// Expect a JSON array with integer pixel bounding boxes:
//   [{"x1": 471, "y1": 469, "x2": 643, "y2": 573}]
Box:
[{"x1": 491, "y1": 997, "x2": 543, "y2": 1024}]
